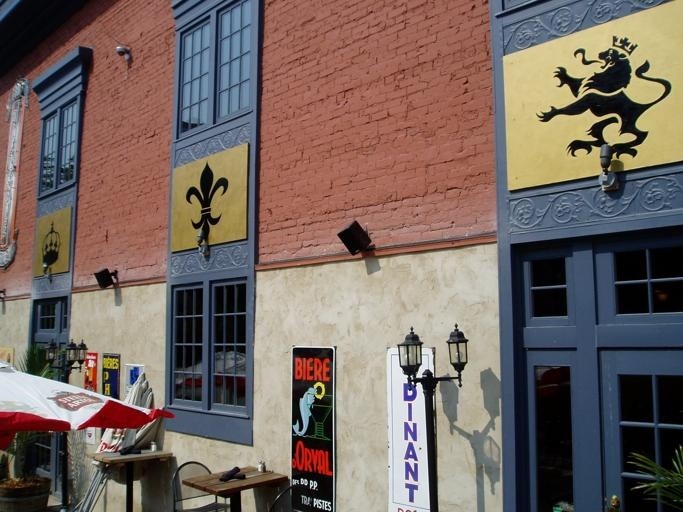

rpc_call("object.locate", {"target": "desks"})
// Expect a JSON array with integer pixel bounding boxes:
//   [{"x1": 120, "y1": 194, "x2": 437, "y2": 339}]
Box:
[
  {"x1": 86, "y1": 447, "x2": 173, "y2": 512},
  {"x1": 181, "y1": 464, "x2": 288, "y2": 512}
]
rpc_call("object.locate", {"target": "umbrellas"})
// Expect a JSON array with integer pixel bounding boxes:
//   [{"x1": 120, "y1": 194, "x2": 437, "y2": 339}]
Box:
[
  {"x1": 75, "y1": 373, "x2": 164, "y2": 512},
  {"x1": 175, "y1": 350, "x2": 245, "y2": 402},
  {"x1": 0, "y1": 362, "x2": 175, "y2": 450}
]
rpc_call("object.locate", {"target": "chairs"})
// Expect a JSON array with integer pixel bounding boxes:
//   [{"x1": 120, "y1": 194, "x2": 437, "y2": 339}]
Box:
[
  {"x1": 267, "y1": 483, "x2": 314, "y2": 512},
  {"x1": 170, "y1": 460, "x2": 235, "y2": 512}
]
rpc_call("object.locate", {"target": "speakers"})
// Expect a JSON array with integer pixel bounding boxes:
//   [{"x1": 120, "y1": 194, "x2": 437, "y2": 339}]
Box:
[
  {"x1": 336, "y1": 221, "x2": 371, "y2": 256},
  {"x1": 94, "y1": 267, "x2": 113, "y2": 289}
]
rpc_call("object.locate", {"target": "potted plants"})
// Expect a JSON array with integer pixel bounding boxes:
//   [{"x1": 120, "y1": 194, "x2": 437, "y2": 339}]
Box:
[{"x1": 0, "y1": 340, "x2": 74, "y2": 512}]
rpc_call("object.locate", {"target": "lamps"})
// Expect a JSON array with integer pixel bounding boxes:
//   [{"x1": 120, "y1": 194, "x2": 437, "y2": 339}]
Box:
[
  {"x1": 194, "y1": 228, "x2": 209, "y2": 259},
  {"x1": 597, "y1": 142, "x2": 620, "y2": 195},
  {"x1": 114, "y1": 45, "x2": 134, "y2": 62},
  {"x1": 41, "y1": 262, "x2": 52, "y2": 283}
]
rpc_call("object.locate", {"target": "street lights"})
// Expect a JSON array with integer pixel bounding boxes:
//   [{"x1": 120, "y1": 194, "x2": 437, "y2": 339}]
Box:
[
  {"x1": 39, "y1": 337, "x2": 86, "y2": 512},
  {"x1": 397, "y1": 323, "x2": 469, "y2": 512}
]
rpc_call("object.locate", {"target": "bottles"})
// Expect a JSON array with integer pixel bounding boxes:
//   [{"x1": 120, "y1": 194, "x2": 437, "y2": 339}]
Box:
[
  {"x1": 257, "y1": 458, "x2": 263, "y2": 471},
  {"x1": 149, "y1": 440, "x2": 156, "y2": 452}
]
